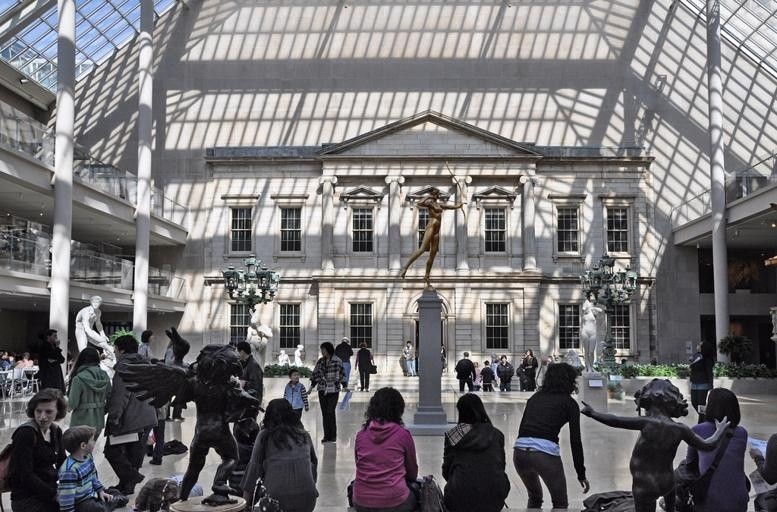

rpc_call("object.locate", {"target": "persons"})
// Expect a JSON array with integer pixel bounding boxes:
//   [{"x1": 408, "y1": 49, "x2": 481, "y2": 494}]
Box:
[
  {"x1": 440, "y1": 343, "x2": 446, "y2": 377},
  {"x1": 1, "y1": 330, "x2": 376, "y2": 512},
  {"x1": 581, "y1": 377, "x2": 733, "y2": 512},
  {"x1": 399, "y1": 187, "x2": 469, "y2": 287},
  {"x1": 402, "y1": 340, "x2": 416, "y2": 376},
  {"x1": 74, "y1": 295, "x2": 116, "y2": 386},
  {"x1": 441, "y1": 392, "x2": 511, "y2": 512},
  {"x1": 578, "y1": 300, "x2": 603, "y2": 374},
  {"x1": 512, "y1": 361, "x2": 589, "y2": 508},
  {"x1": 751, "y1": 434, "x2": 776, "y2": 512},
  {"x1": 455, "y1": 349, "x2": 538, "y2": 392},
  {"x1": 352, "y1": 387, "x2": 419, "y2": 512},
  {"x1": 244, "y1": 308, "x2": 273, "y2": 342},
  {"x1": 687, "y1": 339, "x2": 715, "y2": 424},
  {"x1": 672, "y1": 388, "x2": 751, "y2": 512},
  {"x1": 734, "y1": 267, "x2": 752, "y2": 289}
]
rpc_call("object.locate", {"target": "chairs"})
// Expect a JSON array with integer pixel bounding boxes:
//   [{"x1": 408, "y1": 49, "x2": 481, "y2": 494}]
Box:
[{"x1": 0, "y1": 365, "x2": 40, "y2": 399}]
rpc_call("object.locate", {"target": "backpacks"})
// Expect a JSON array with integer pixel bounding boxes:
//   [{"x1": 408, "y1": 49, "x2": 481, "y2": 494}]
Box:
[{"x1": 0, "y1": 443, "x2": 14, "y2": 493}]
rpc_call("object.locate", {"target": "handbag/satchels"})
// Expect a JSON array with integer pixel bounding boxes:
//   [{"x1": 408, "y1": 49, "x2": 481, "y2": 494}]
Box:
[
  {"x1": 674, "y1": 460, "x2": 707, "y2": 509},
  {"x1": 370, "y1": 365, "x2": 377, "y2": 374},
  {"x1": 341, "y1": 393, "x2": 352, "y2": 410},
  {"x1": 148, "y1": 440, "x2": 187, "y2": 456},
  {"x1": 411, "y1": 475, "x2": 446, "y2": 512},
  {"x1": 253, "y1": 497, "x2": 282, "y2": 511}
]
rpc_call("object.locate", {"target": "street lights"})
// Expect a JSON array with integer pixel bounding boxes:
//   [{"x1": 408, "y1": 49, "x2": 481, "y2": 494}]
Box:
[{"x1": 579, "y1": 252, "x2": 637, "y2": 371}]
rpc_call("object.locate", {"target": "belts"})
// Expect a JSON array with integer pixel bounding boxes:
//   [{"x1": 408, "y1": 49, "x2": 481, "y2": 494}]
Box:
[{"x1": 518, "y1": 446, "x2": 539, "y2": 452}]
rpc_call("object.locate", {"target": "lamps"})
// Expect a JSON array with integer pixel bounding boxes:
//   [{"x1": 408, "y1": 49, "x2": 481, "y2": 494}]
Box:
[{"x1": 580, "y1": 252, "x2": 637, "y2": 308}]
